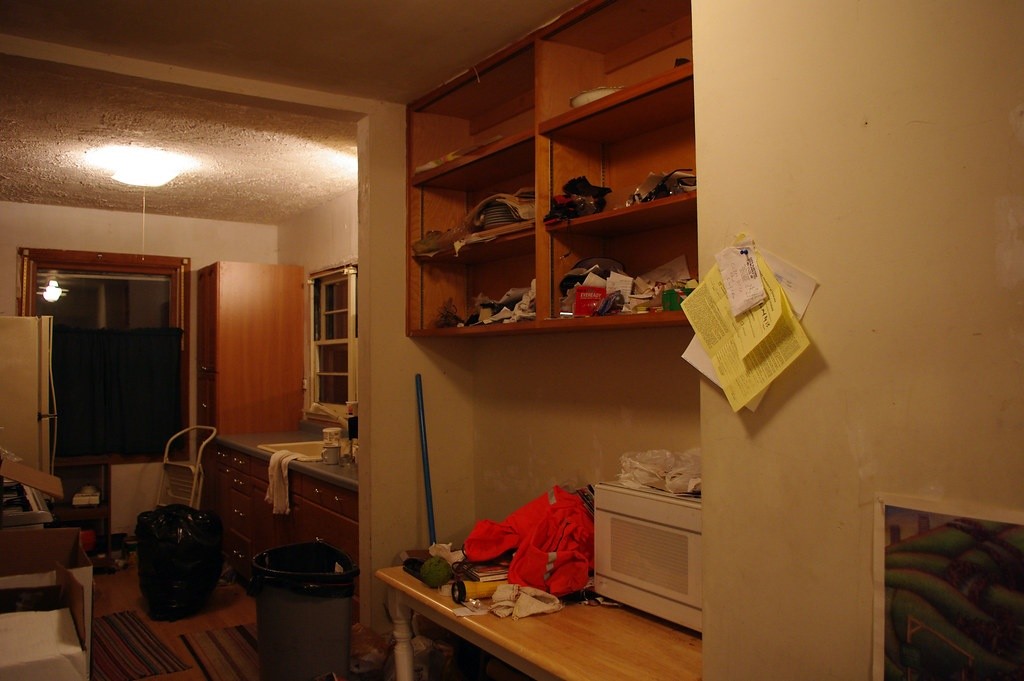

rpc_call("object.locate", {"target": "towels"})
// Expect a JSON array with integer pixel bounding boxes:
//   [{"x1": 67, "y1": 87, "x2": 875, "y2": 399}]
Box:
[{"x1": 263, "y1": 449, "x2": 307, "y2": 514}]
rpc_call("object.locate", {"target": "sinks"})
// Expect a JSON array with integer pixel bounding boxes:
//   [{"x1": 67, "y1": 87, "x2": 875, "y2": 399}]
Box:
[{"x1": 257, "y1": 441, "x2": 338, "y2": 461}]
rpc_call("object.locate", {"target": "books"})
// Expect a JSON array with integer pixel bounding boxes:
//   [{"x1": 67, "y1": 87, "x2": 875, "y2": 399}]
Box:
[{"x1": 463, "y1": 564, "x2": 508, "y2": 582}]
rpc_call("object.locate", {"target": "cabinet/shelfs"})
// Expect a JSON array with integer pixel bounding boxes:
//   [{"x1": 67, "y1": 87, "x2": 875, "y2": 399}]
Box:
[
  {"x1": 198, "y1": 443, "x2": 360, "y2": 625},
  {"x1": 196, "y1": 260, "x2": 305, "y2": 511},
  {"x1": 404, "y1": 0, "x2": 698, "y2": 336},
  {"x1": 53, "y1": 460, "x2": 119, "y2": 572}
]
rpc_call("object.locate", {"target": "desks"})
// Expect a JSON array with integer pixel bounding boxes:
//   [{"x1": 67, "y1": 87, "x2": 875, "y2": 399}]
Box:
[{"x1": 373, "y1": 562, "x2": 702, "y2": 681}]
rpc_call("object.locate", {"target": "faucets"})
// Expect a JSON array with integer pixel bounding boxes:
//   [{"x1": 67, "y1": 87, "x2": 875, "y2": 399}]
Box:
[{"x1": 309, "y1": 401, "x2": 348, "y2": 439}]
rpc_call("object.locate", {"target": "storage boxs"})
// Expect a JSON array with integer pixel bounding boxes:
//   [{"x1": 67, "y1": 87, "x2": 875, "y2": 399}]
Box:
[{"x1": 1, "y1": 526, "x2": 94, "y2": 680}]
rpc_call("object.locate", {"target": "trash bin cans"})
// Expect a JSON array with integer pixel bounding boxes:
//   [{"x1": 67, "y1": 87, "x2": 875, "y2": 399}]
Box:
[{"x1": 251, "y1": 540, "x2": 357, "y2": 681}]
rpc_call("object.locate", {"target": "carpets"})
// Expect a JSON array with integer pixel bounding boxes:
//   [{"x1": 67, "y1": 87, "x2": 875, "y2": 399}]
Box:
[
  {"x1": 180, "y1": 623, "x2": 262, "y2": 681},
  {"x1": 90, "y1": 610, "x2": 193, "y2": 681}
]
